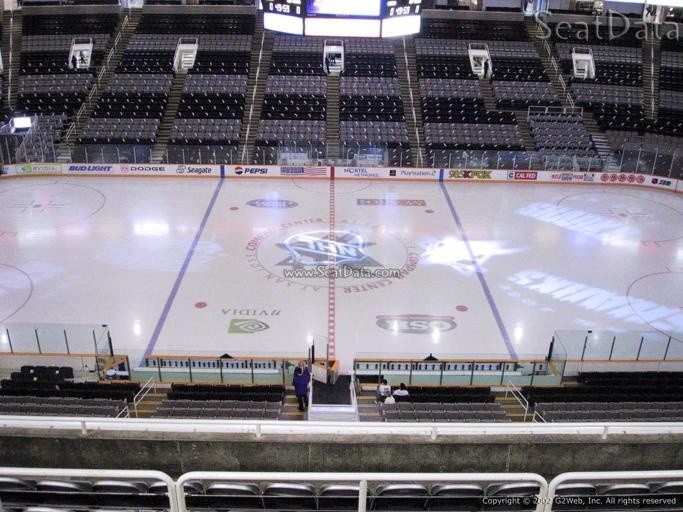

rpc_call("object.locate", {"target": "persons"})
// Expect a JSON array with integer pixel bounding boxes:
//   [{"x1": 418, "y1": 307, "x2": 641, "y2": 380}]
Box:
[
  {"x1": 484, "y1": 58, "x2": 489, "y2": 78},
  {"x1": 425, "y1": 353, "x2": 436, "y2": 360},
  {"x1": 292, "y1": 361, "x2": 310, "y2": 412},
  {"x1": 325, "y1": 55, "x2": 331, "y2": 73},
  {"x1": 374, "y1": 379, "x2": 409, "y2": 404},
  {"x1": 71, "y1": 51, "x2": 86, "y2": 69}
]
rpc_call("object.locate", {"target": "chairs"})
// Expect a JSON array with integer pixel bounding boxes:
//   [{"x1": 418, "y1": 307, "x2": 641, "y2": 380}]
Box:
[
  {"x1": 0, "y1": 475, "x2": 683, "y2": 511},
  {"x1": 1, "y1": 364, "x2": 621, "y2": 422}
]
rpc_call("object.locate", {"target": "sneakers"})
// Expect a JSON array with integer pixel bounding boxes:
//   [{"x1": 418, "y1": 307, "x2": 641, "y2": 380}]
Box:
[{"x1": 299, "y1": 403, "x2": 308, "y2": 411}]
